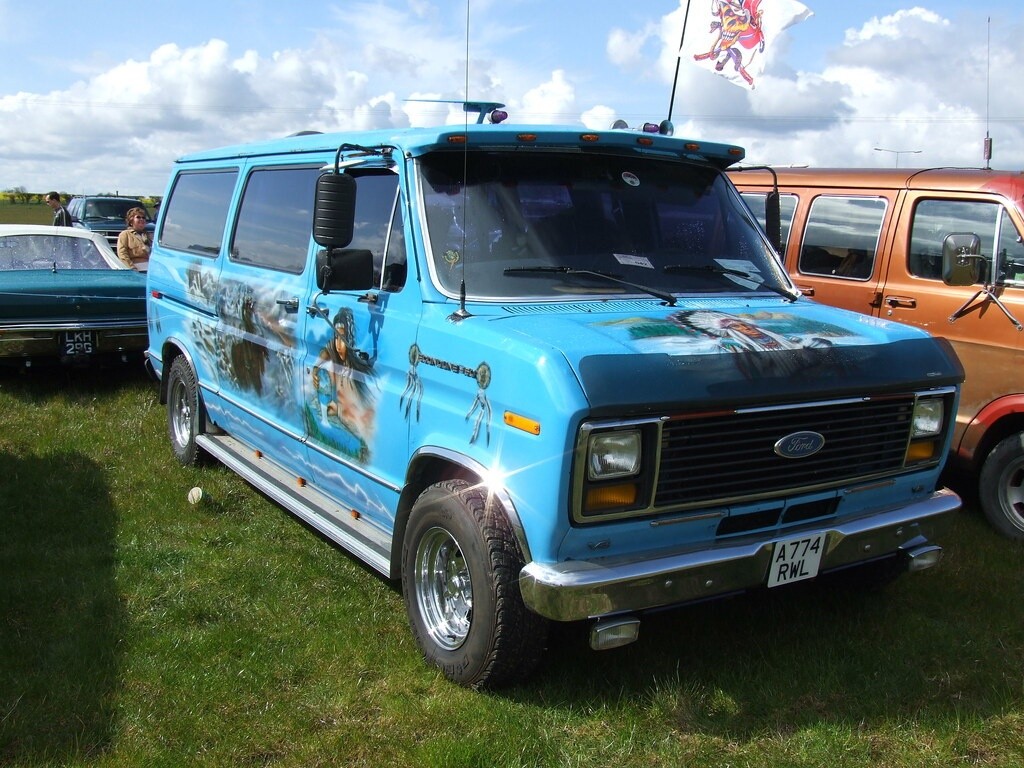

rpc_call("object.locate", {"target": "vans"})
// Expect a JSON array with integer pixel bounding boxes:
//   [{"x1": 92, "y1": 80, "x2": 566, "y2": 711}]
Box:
[
  {"x1": 143, "y1": 102, "x2": 969, "y2": 687},
  {"x1": 717, "y1": 163, "x2": 1023, "y2": 543}
]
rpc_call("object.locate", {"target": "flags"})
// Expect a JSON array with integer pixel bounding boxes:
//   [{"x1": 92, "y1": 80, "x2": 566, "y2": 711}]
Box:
[{"x1": 678, "y1": 0, "x2": 814, "y2": 91}]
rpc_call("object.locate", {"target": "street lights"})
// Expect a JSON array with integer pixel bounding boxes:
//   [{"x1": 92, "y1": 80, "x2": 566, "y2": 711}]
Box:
[{"x1": 874, "y1": 148, "x2": 924, "y2": 170}]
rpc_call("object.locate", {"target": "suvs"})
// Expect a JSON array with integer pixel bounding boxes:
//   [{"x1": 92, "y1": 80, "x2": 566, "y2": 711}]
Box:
[{"x1": 67, "y1": 195, "x2": 158, "y2": 261}]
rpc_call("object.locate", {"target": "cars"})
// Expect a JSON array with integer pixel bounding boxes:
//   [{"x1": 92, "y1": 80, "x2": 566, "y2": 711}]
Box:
[{"x1": 1, "y1": 225, "x2": 151, "y2": 374}]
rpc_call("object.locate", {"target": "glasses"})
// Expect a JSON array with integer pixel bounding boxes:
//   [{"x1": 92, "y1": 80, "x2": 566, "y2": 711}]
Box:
[
  {"x1": 46, "y1": 199, "x2": 52, "y2": 204},
  {"x1": 133, "y1": 215, "x2": 146, "y2": 220}
]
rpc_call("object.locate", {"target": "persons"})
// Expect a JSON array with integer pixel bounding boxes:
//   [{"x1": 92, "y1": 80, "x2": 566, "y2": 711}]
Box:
[
  {"x1": 46, "y1": 192, "x2": 77, "y2": 260},
  {"x1": 117, "y1": 208, "x2": 152, "y2": 274}
]
